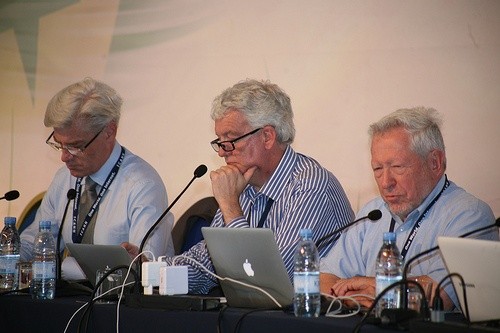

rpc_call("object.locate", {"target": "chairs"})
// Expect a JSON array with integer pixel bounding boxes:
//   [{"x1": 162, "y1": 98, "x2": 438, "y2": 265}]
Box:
[
  {"x1": 172, "y1": 196, "x2": 219, "y2": 255},
  {"x1": 15, "y1": 191, "x2": 46, "y2": 235}
]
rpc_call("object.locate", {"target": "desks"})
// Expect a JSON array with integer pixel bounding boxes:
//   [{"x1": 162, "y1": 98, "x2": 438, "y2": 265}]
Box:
[{"x1": 0, "y1": 295, "x2": 500, "y2": 333}]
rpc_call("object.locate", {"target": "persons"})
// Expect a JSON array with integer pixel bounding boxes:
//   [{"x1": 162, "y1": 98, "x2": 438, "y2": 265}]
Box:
[
  {"x1": 319, "y1": 107, "x2": 499, "y2": 308},
  {"x1": 121, "y1": 78, "x2": 355, "y2": 295},
  {"x1": 19, "y1": 78, "x2": 176, "y2": 268}
]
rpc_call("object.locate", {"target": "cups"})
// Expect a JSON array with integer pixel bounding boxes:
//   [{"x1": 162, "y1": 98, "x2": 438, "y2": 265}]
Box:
[
  {"x1": 95, "y1": 268, "x2": 123, "y2": 304},
  {"x1": 16, "y1": 261, "x2": 32, "y2": 290}
]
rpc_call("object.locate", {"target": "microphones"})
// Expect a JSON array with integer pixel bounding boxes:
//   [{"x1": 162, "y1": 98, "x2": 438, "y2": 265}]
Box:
[
  {"x1": 126, "y1": 164, "x2": 221, "y2": 311},
  {"x1": 380, "y1": 218, "x2": 500, "y2": 322},
  {"x1": 51, "y1": 186, "x2": 96, "y2": 297},
  {"x1": 296, "y1": 209, "x2": 382, "y2": 313},
  {"x1": 0, "y1": 190, "x2": 20, "y2": 201}
]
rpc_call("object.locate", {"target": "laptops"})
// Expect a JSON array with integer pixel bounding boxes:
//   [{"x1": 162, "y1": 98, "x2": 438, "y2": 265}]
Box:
[
  {"x1": 66, "y1": 244, "x2": 142, "y2": 301},
  {"x1": 436, "y1": 237, "x2": 500, "y2": 329},
  {"x1": 199, "y1": 226, "x2": 296, "y2": 311}
]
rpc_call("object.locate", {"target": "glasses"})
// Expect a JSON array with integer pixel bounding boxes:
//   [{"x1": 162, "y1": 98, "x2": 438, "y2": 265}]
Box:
[
  {"x1": 210, "y1": 126, "x2": 276, "y2": 153},
  {"x1": 46, "y1": 127, "x2": 105, "y2": 156}
]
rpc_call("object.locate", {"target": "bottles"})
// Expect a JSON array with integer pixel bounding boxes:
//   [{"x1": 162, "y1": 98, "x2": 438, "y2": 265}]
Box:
[
  {"x1": 293, "y1": 228, "x2": 321, "y2": 319},
  {"x1": 31, "y1": 221, "x2": 56, "y2": 302},
  {"x1": 375, "y1": 233, "x2": 403, "y2": 319},
  {"x1": 0, "y1": 217, "x2": 22, "y2": 290}
]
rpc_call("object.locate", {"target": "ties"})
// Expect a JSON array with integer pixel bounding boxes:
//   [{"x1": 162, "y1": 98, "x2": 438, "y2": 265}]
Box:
[{"x1": 78, "y1": 176, "x2": 102, "y2": 245}]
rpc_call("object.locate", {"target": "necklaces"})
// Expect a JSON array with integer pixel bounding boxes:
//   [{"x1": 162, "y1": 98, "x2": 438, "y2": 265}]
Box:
[{"x1": 246, "y1": 200, "x2": 272, "y2": 229}]
